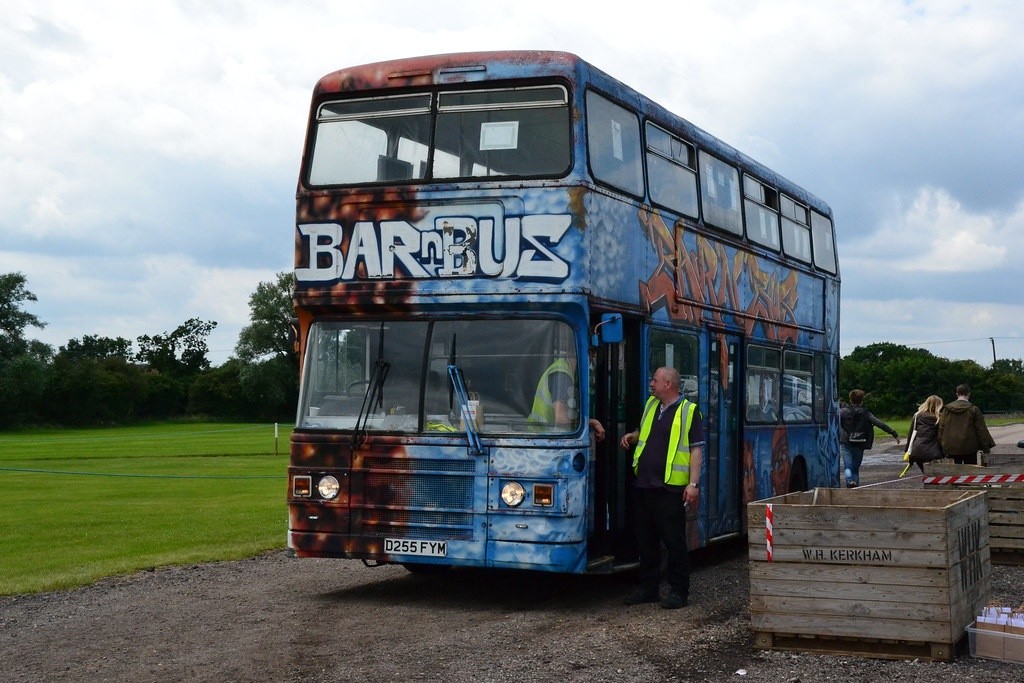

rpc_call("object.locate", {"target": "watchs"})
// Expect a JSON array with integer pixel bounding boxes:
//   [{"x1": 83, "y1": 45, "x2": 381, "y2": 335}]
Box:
[{"x1": 688, "y1": 482, "x2": 700, "y2": 488}]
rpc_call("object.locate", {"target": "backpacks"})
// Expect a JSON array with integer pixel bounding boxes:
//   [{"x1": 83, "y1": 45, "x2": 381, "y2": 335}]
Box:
[{"x1": 841, "y1": 404, "x2": 874, "y2": 450}]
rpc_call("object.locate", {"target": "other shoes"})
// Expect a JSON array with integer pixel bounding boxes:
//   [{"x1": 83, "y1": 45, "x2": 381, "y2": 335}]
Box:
[
  {"x1": 660, "y1": 591, "x2": 689, "y2": 609},
  {"x1": 624, "y1": 590, "x2": 660, "y2": 604}
]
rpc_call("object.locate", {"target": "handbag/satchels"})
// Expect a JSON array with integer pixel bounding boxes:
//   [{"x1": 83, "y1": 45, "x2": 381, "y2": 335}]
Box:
[
  {"x1": 907, "y1": 416, "x2": 917, "y2": 455},
  {"x1": 976, "y1": 601, "x2": 1024, "y2": 662}
]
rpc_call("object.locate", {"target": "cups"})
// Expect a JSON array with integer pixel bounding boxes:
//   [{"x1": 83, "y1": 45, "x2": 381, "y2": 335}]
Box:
[
  {"x1": 309, "y1": 407, "x2": 320, "y2": 416},
  {"x1": 396, "y1": 406, "x2": 406, "y2": 415}
]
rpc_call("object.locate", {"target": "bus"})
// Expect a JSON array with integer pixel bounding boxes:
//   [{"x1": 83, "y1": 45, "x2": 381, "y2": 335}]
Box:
[{"x1": 286, "y1": 51, "x2": 842, "y2": 584}]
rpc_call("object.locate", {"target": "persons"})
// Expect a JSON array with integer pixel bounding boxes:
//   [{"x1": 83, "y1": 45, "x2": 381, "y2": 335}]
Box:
[
  {"x1": 840, "y1": 389, "x2": 900, "y2": 488},
  {"x1": 528, "y1": 341, "x2": 605, "y2": 442},
  {"x1": 938, "y1": 384, "x2": 996, "y2": 465},
  {"x1": 620, "y1": 366, "x2": 705, "y2": 608},
  {"x1": 904, "y1": 395, "x2": 946, "y2": 474}
]
